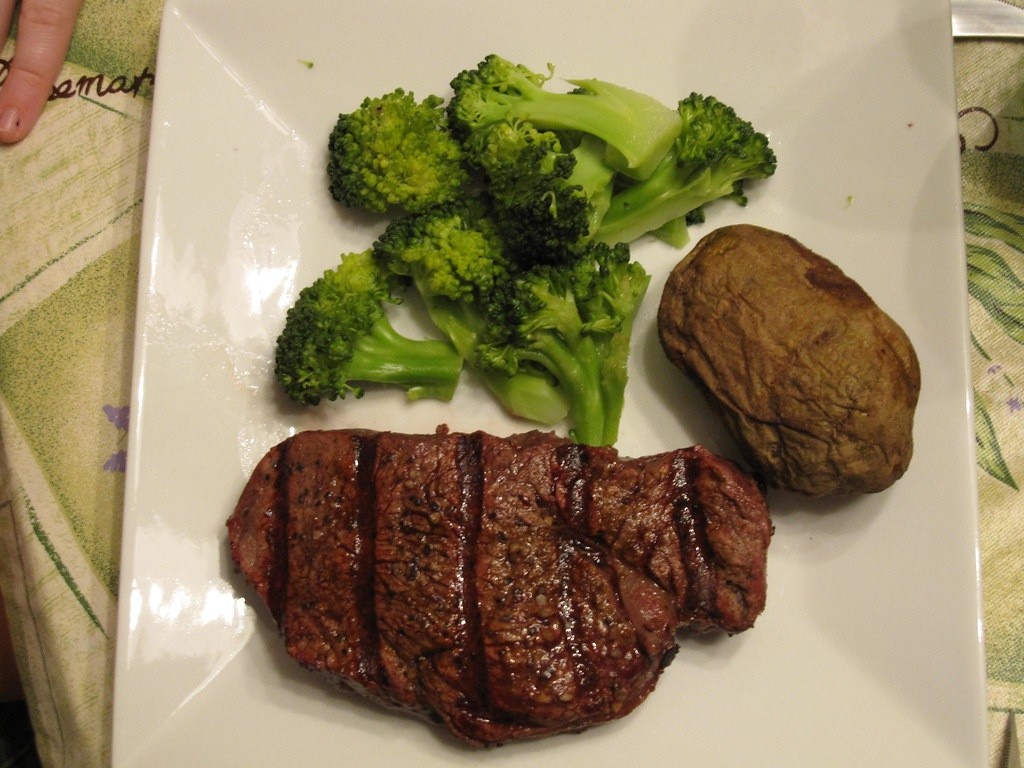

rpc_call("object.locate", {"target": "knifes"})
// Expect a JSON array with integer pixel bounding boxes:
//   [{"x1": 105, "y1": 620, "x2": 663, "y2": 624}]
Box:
[{"x1": 950, "y1": 0, "x2": 1024, "y2": 37}]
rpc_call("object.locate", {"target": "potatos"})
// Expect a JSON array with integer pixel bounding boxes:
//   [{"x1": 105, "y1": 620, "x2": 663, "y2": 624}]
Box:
[{"x1": 657, "y1": 222, "x2": 919, "y2": 500}]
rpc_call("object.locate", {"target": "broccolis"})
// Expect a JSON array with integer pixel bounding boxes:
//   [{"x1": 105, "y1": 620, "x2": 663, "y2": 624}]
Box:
[{"x1": 272, "y1": 55, "x2": 783, "y2": 446}]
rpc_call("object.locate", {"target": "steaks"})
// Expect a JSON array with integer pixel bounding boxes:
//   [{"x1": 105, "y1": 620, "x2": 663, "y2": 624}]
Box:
[{"x1": 226, "y1": 424, "x2": 771, "y2": 751}]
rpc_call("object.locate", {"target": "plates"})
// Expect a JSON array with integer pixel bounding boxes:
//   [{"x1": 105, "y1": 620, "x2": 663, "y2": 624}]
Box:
[{"x1": 111, "y1": 0, "x2": 987, "y2": 768}]
